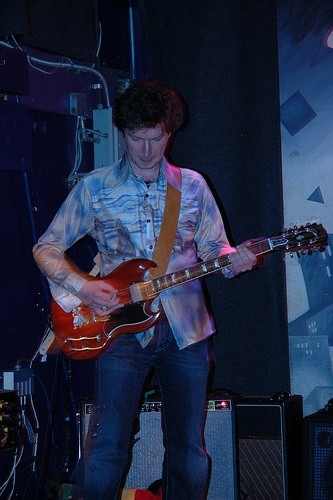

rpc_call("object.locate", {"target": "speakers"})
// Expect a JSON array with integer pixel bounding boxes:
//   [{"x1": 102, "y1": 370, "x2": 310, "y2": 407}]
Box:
[
  {"x1": 80, "y1": 397, "x2": 238, "y2": 500},
  {"x1": 237, "y1": 394, "x2": 303, "y2": 500},
  {"x1": 307, "y1": 423, "x2": 333, "y2": 500}
]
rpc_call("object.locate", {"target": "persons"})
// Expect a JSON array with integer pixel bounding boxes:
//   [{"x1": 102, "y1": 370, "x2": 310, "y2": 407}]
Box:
[{"x1": 31, "y1": 78, "x2": 257, "y2": 500}]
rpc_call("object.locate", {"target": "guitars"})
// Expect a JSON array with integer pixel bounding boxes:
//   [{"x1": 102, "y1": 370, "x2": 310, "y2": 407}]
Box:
[{"x1": 50, "y1": 222, "x2": 328, "y2": 362}]
